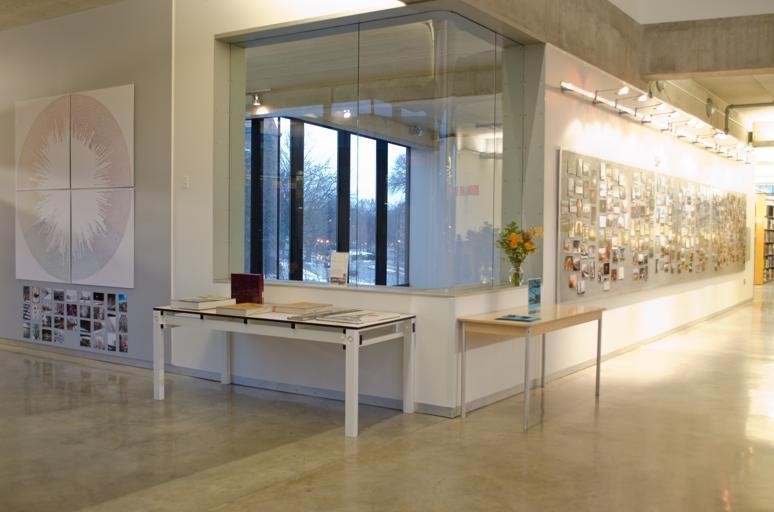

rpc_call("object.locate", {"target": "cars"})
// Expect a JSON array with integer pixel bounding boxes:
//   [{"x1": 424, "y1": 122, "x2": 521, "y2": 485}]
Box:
[{"x1": 312, "y1": 246, "x2": 405, "y2": 285}]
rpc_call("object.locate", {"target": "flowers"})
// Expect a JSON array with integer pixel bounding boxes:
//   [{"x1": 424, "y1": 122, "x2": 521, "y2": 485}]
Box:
[{"x1": 494, "y1": 219, "x2": 544, "y2": 289}]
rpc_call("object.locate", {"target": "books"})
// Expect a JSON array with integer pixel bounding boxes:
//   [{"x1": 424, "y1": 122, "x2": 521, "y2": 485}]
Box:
[
  {"x1": 273, "y1": 300, "x2": 333, "y2": 314},
  {"x1": 171, "y1": 294, "x2": 237, "y2": 310},
  {"x1": 215, "y1": 302, "x2": 273, "y2": 316},
  {"x1": 315, "y1": 307, "x2": 400, "y2": 324}
]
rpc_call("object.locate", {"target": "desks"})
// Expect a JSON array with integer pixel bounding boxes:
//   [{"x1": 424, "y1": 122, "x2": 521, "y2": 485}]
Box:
[
  {"x1": 457, "y1": 302, "x2": 608, "y2": 433},
  {"x1": 150, "y1": 300, "x2": 418, "y2": 440}
]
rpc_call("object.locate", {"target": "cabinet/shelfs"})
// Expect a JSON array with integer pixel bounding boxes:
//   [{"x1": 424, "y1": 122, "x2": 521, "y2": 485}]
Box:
[{"x1": 754, "y1": 194, "x2": 773, "y2": 286}]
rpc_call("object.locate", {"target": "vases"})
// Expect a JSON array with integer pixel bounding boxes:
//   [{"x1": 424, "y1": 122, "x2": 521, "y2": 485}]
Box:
[{"x1": 508, "y1": 267, "x2": 524, "y2": 288}]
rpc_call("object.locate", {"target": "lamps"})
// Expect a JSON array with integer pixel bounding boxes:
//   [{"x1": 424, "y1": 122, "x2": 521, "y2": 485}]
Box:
[
  {"x1": 590, "y1": 86, "x2": 755, "y2": 165},
  {"x1": 250, "y1": 92, "x2": 263, "y2": 107},
  {"x1": 412, "y1": 124, "x2": 424, "y2": 136}
]
